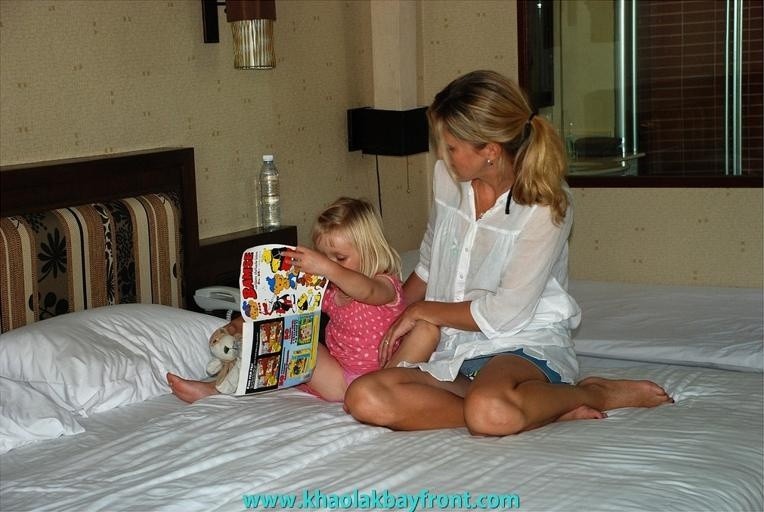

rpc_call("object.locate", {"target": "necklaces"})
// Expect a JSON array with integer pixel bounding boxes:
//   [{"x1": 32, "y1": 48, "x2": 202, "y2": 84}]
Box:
[{"x1": 474, "y1": 186, "x2": 504, "y2": 221}]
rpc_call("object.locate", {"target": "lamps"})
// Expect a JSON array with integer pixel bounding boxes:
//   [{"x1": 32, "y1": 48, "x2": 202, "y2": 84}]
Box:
[{"x1": 196, "y1": 0, "x2": 280, "y2": 75}]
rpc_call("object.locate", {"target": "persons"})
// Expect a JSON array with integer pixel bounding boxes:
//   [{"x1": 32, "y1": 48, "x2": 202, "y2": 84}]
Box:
[
  {"x1": 206, "y1": 317, "x2": 244, "y2": 394},
  {"x1": 166, "y1": 197, "x2": 406, "y2": 402},
  {"x1": 341, "y1": 68, "x2": 675, "y2": 436}
]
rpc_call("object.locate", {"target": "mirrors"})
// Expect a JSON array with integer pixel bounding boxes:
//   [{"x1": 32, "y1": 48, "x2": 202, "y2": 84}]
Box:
[{"x1": 518, "y1": 2, "x2": 557, "y2": 108}]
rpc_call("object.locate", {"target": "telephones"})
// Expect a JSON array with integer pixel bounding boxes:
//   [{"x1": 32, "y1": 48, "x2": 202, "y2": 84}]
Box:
[{"x1": 193, "y1": 286, "x2": 241, "y2": 313}]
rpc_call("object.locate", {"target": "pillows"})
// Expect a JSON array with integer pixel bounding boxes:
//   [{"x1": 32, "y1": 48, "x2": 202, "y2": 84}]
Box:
[
  {"x1": 0, "y1": 374, "x2": 87, "y2": 458},
  {"x1": 0, "y1": 299, "x2": 247, "y2": 418}
]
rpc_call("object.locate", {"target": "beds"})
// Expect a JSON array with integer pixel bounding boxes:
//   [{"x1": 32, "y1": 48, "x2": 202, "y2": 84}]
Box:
[{"x1": 0, "y1": 142, "x2": 764, "y2": 512}]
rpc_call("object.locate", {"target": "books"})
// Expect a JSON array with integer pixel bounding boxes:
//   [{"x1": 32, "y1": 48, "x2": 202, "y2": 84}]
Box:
[{"x1": 236, "y1": 242, "x2": 329, "y2": 396}]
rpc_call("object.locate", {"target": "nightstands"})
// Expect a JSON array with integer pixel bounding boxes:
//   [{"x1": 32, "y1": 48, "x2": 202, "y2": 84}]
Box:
[{"x1": 195, "y1": 226, "x2": 302, "y2": 324}]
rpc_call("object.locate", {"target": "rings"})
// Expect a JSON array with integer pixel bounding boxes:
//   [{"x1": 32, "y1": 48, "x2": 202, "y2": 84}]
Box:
[{"x1": 384, "y1": 339, "x2": 389, "y2": 346}]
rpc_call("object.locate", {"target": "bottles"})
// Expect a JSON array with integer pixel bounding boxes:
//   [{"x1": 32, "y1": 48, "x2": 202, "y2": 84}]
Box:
[{"x1": 258, "y1": 153, "x2": 282, "y2": 232}]
